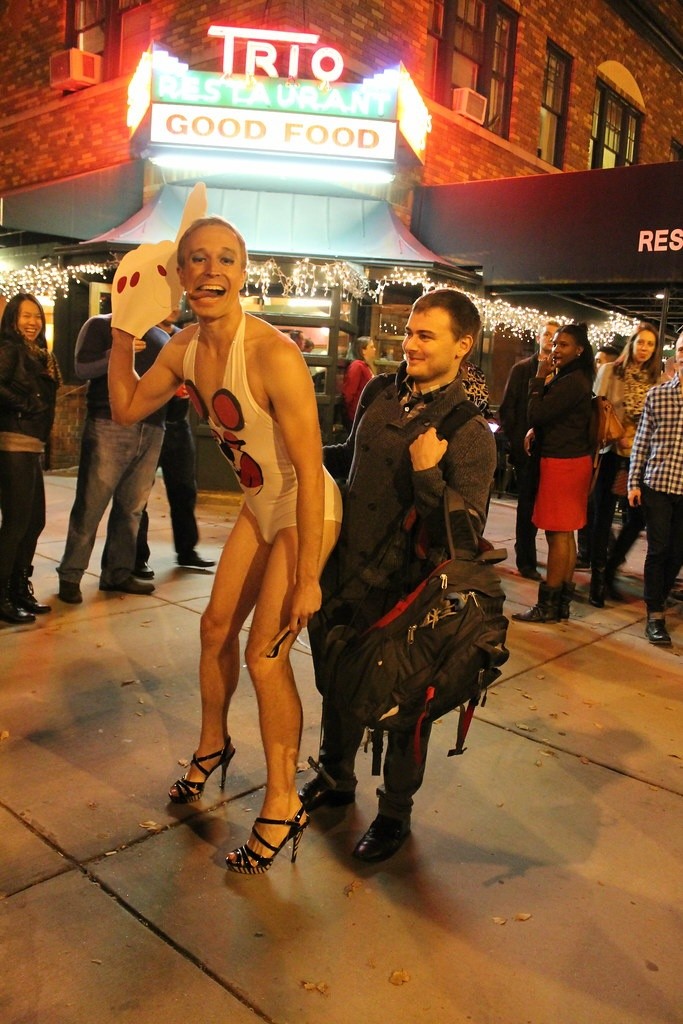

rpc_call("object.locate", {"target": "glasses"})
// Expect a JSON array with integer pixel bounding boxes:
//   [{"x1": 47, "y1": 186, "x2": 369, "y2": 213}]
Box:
[{"x1": 596, "y1": 343, "x2": 621, "y2": 353}]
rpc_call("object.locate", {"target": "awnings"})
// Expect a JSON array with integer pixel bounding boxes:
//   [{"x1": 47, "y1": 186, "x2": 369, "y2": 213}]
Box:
[
  {"x1": 78, "y1": 186, "x2": 460, "y2": 269},
  {"x1": 0, "y1": 158, "x2": 143, "y2": 241}
]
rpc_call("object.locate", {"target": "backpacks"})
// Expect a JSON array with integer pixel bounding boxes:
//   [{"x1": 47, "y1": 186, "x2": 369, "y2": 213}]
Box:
[{"x1": 316, "y1": 485, "x2": 512, "y2": 775}]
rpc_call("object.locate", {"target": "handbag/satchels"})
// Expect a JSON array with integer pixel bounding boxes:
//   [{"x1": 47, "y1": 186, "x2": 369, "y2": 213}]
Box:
[{"x1": 594, "y1": 394, "x2": 625, "y2": 468}]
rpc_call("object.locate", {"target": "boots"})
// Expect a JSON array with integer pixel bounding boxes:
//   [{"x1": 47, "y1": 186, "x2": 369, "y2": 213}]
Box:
[
  {"x1": 559, "y1": 580, "x2": 577, "y2": 619},
  {"x1": 511, "y1": 582, "x2": 560, "y2": 624}
]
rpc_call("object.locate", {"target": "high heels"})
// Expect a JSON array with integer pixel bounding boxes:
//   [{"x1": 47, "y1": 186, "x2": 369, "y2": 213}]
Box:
[
  {"x1": 168, "y1": 735, "x2": 236, "y2": 804},
  {"x1": 226, "y1": 802, "x2": 311, "y2": 874}
]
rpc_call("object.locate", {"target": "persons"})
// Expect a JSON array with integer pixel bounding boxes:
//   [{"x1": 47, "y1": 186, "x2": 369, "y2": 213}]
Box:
[
  {"x1": 57, "y1": 314, "x2": 171, "y2": 604},
  {"x1": 511, "y1": 323, "x2": 596, "y2": 623},
  {"x1": 588, "y1": 322, "x2": 660, "y2": 608},
  {"x1": 337, "y1": 337, "x2": 375, "y2": 429},
  {"x1": 303, "y1": 289, "x2": 496, "y2": 862},
  {"x1": 108, "y1": 180, "x2": 346, "y2": 875},
  {"x1": 628, "y1": 330, "x2": 683, "y2": 645},
  {"x1": 0, "y1": 294, "x2": 61, "y2": 624},
  {"x1": 575, "y1": 346, "x2": 618, "y2": 569},
  {"x1": 503, "y1": 322, "x2": 555, "y2": 579}
]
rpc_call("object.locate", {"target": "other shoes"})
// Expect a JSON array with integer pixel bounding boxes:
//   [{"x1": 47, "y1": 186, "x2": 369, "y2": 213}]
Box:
[
  {"x1": 520, "y1": 569, "x2": 542, "y2": 578},
  {"x1": 589, "y1": 569, "x2": 606, "y2": 607},
  {"x1": 351, "y1": 813, "x2": 411, "y2": 862},
  {"x1": 0, "y1": 592, "x2": 36, "y2": 624},
  {"x1": 6, "y1": 580, "x2": 52, "y2": 613},
  {"x1": 574, "y1": 552, "x2": 591, "y2": 568},
  {"x1": 99, "y1": 575, "x2": 156, "y2": 594},
  {"x1": 177, "y1": 551, "x2": 215, "y2": 567},
  {"x1": 644, "y1": 619, "x2": 671, "y2": 645},
  {"x1": 59, "y1": 579, "x2": 83, "y2": 603},
  {"x1": 133, "y1": 561, "x2": 155, "y2": 577},
  {"x1": 296, "y1": 774, "x2": 359, "y2": 813}
]
